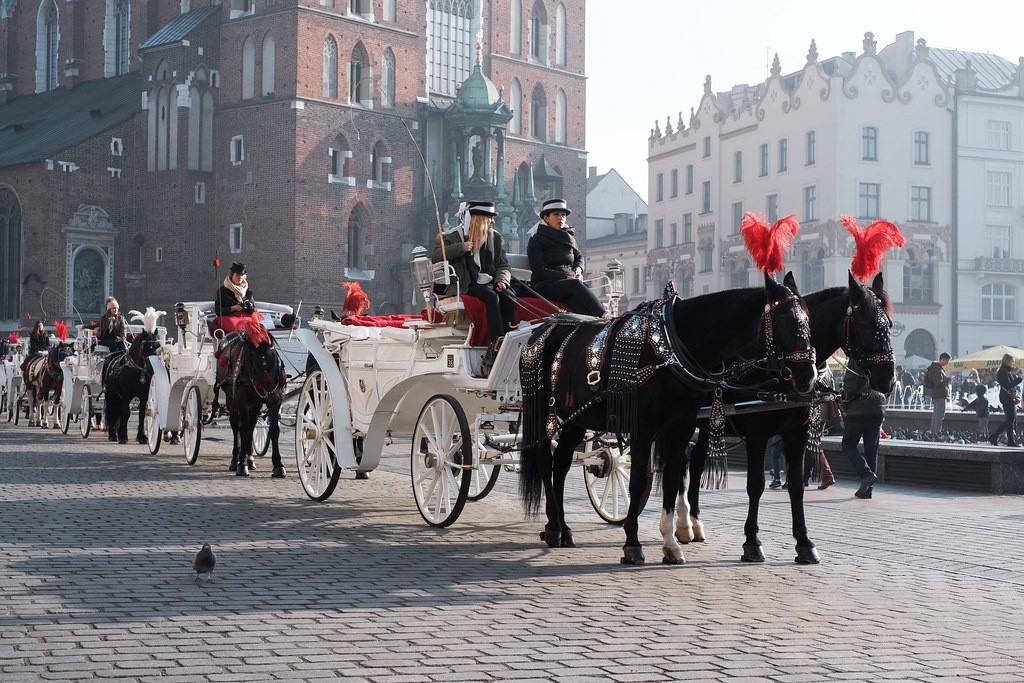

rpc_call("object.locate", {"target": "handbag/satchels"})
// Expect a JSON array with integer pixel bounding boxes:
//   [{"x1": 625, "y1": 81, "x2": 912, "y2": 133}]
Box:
[{"x1": 1011, "y1": 391, "x2": 1022, "y2": 404}]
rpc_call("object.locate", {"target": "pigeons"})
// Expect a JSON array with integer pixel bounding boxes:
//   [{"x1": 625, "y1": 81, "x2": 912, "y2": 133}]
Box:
[{"x1": 192, "y1": 543, "x2": 216, "y2": 583}]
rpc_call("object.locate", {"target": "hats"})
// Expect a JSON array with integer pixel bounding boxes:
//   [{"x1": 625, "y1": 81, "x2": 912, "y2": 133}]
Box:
[
  {"x1": 3, "y1": 337, "x2": 9, "y2": 343},
  {"x1": 539, "y1": 199, "x2": 571, "y2": 219},
  {"x1": 106, "y1": 297, "x2": 119, "y2": 310},
  {"x1": 228, "y1": 261, "x2": 247, "y2": 274},
  {"x1": 462, "y1": 201, "x2": 498, "y2": 217}
]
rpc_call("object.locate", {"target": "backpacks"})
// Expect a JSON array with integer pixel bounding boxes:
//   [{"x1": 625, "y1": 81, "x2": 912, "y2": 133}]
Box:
[{"x1": 924, "y1": 364, "x2": 943, "y2": 389}]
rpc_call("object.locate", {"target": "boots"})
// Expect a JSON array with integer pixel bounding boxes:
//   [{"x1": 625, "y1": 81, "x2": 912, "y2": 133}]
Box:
[{"x1": 484, "y1": 342, "x2": 500, "y2": 365}]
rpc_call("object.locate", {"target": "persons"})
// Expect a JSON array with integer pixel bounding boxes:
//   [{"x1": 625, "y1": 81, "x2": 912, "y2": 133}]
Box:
[
  {"x1": 311, "y1": 304, "x2": 325, "y2": 320},
  {"x1": 896, "y1": 365, "x2": 925, "y2": 409},
  {"x1": 840, "y1": 358, "x2": 886, "y2": 499},
  {"x1": 0, "y1": 337, "x2": 10, "y2": 363},
  {"x1": 931, "y1": 352, "x2": 951, "y2": 441},
  {"x1": 804, "y1": 361, "x2": 839, "y2": 490},
  {"x1": 947, "y1": 376, "x2": 957, "y2": 401},
  {"x1": 768, "y1": 434, "x2": 789, "y2": 488},
  {"x1": 82, "y1": 296, "x2": 128, "y2": 352},
  {"x1": 472, "y1": 142, "x2": 483, "y2": 176},
  {"x1": 434, "y1": 200, "x2": 517, "y2": 347},
  {"x1": 214, "y1": 262, "x2": 257, "y2": 316},
  {"x1": 527, "y1": 198, "x2": 610, "y2": 319},
  {"x1": 30, "y1": 321, "x2": 51, "y2": 352},
  {"x1": 959, "y1": 368, "x2": 990, "y2": 438},
  {"x1": 988, "y1": 353, "x2": 1024, "y2": 447}
]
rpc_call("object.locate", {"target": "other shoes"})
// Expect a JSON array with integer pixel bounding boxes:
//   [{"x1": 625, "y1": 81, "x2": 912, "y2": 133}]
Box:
[
  {"x1": 768, "y1": 479, "x2": 781, "y2": 488},
  {"x1": 1006, "y1": 440, "x2": 1021, "y2": 448},
  {"x1": 855, "y1": 473, "x2": 877, "y2": 496},
  {"x1": 988, "y1": 434, "x2": 997, "y2": 446},
  {"x1": 818, "y1": 478, "x2": 835, "y2": 490},
  {"x1": 781, "y1": 483, "x2": 789, "y2": 489}
]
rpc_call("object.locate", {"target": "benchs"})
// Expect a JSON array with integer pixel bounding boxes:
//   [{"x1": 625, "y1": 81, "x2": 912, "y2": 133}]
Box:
[
  {"x1": 198, "y1": 321, "x2": 299, "y2": 354},
  {"x1": 437, "y1": 267, "x2": 568, "y2": 329}
]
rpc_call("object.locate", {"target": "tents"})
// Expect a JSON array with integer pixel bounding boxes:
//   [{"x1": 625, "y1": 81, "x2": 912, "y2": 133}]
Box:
[{"x1": 962, "y1": 345, "x2": 1024, "y2": 369}]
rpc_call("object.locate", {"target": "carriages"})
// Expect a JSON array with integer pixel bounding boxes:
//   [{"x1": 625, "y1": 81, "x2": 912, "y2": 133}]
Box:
[
  {"x1": 143, "y1": 281, "x2": 372, "y2": 482},
  {"x1": 291, "y1": 247, "x2": 896, "y2": 568},
  {"x1": 0, "y1": 305, "x2": 298, "y2": 446}
]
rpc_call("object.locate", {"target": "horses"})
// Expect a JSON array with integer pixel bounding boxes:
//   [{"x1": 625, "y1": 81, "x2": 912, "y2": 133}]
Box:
[
  {"x1": 20, "y1": 320, "x2": 75, "y2": 429},
  {"x1": 216, "y1": 312, "x2": 287, "y2": 478},
  {"x1": 101, "y1": 307, "x2": 167, "y2": 443},
  {"x1": 518, "y1": 212, "x2": 817, "y2": 565},
  {"x1": 662, "y1": 214, "x2": 897, "y2": 564}
]
